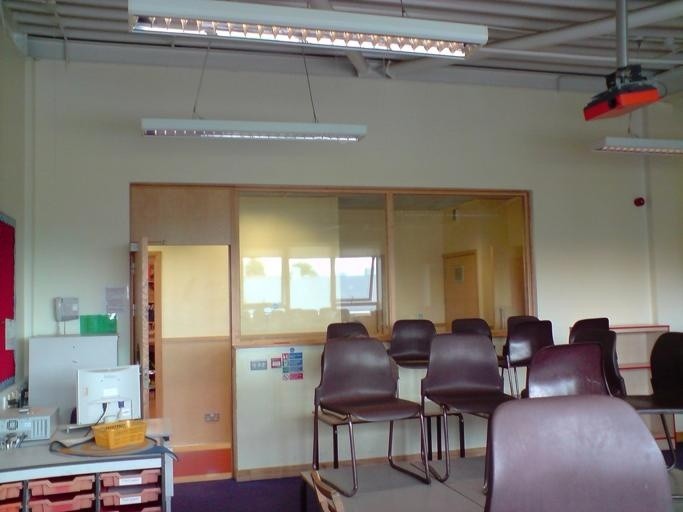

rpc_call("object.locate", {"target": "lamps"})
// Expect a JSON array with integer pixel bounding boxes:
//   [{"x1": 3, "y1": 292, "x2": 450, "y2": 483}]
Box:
[
  {"x1": 138, "y1": 40, "x2": 369, "y2": 139},
  {"x1": 123, "y1": 3, "x2": 489, "y2": 54},
  {"x1": 597, "y1": 111, "x2": 683, "y2": 156}
]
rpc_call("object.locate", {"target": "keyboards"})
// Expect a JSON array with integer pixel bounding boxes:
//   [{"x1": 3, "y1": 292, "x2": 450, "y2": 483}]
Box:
[{"x1": 56, "y1": 423, "x2": 81, "y2": 433}]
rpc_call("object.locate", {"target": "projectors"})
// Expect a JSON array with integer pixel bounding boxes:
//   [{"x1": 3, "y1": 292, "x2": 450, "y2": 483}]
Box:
[{"x1": 583, "y1": 81, "x2": 667, "y2": 123}]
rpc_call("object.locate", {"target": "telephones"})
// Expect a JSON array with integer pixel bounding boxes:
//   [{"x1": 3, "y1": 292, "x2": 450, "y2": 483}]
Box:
[{"x1": 55, "y1": 297, "x2": 79, "y2": 321}]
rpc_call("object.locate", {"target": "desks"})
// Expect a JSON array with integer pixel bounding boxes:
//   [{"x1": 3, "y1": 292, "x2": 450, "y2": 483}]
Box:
[{"x1": 2, "y1": 431, "x2": 175, "y2": 511}]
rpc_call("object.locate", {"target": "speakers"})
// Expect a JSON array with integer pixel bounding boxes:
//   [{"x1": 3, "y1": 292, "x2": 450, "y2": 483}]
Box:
[{"x1": 0, "y1": 407, "x2": 60, "y2": 441}]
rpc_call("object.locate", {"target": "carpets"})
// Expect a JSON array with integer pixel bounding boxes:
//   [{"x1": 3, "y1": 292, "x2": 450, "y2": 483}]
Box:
[{"x1": 175, "y1": 473, "x2": 323, "y2": 509}]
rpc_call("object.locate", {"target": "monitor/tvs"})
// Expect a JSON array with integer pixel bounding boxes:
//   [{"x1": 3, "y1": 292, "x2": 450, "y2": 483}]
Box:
[{"x1": 76, "y1": 365, "x2": 142, "y2": 428}]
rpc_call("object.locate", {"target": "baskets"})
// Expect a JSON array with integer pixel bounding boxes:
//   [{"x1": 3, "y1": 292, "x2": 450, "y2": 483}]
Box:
[{"x1": 91, "y1": 419, "x2": 147, "y2": 450}]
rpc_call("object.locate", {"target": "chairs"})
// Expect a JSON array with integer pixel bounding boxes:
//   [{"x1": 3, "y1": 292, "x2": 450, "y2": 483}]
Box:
[
  {"x1": 648, "y1": 329, "x2": 683, "y2": 405},
  {"x1": 522, "y1": 340, "x2": 614, "y2": 400},
  {"x1": 418, "y1": 332, "x2": 520, "y2": 483},
  {"x1": 487, "y1": 391, "x2": 673, "y2": 511},
  {"x1": 309, "y1": 333, "x2": 433, "y2": 497},
  {"x1": 319, "y1": 322, "x2": 398, "y2": 472},
  {"x1": 495, "y1": 315, "x2": 629, "y2": 396},
  {"x1": 449, "y1": 316, "x2": 494, "y2": 453},
  {"x1": 388, "y1": 318, "x2": 466, "y2": 486}
]
